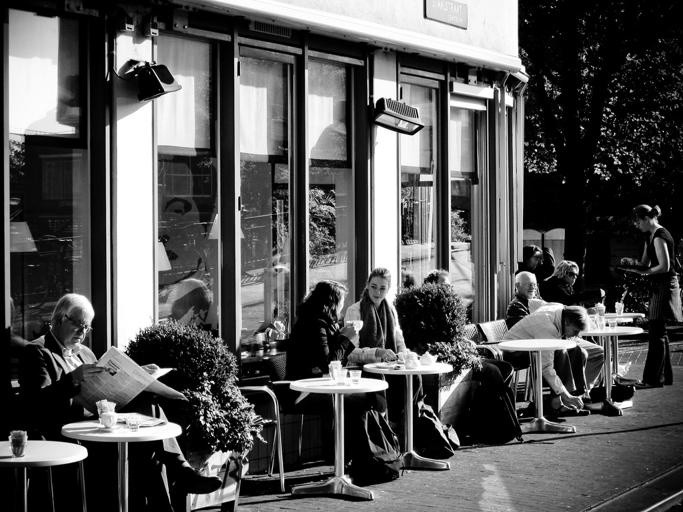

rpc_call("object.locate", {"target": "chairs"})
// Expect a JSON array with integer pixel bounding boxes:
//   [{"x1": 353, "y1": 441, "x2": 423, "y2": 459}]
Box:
[
  {"x1": 263, "y1": 352, "x2": 326, "y2": 478},
  {"x1": 460, "y1": 318, "x2": 532, "y2": 418}
]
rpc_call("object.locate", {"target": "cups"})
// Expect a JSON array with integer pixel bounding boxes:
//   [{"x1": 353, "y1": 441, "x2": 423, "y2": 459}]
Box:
[
  {"x1": 401, "y1": 350, "x2": 435, "y2": 370},
  {"x1": 327, "y1": 360, "x2": 362, "y2": 387},
  {"x1": 124, "y1": 416, "x2": 140, "y2": 433},
  {"x1": 99, "y1": 412, "x2": 116, "y2": 433},
  {"x1": 597, "y1": 306, "x2": 604, "y2": 316},
  {"x1": 7, "y1": 435, "x2": 27, "y2": 457},
  {"x1": 595, "y1": 317, "x2": 616, "y2": 330},
  {"x1": 615, "y1": 305, "x2": 622, "y2": 317}
]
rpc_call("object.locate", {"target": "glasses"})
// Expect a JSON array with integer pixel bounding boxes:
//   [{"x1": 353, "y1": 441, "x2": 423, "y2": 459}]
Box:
[
  {"x1": 565, "y1": 272, "x2": 581, "y2": 280},
  {"x1": 65, "y1": 315, "x2": 93, "y2": 334}
]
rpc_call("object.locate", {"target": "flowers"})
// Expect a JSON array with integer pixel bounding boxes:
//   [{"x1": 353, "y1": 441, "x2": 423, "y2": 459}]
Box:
[{"x1": 252, "y1": 317, "x2": 289, "y2": 358}]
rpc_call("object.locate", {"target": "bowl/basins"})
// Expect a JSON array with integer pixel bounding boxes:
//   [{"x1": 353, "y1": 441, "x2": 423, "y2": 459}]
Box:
[{"x1": 346, "y1": 320, "x2": 363, "y2": 332}]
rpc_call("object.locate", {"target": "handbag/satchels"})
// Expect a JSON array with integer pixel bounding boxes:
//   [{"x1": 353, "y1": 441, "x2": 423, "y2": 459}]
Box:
[
  {"x1": 590, "y1": 377, "x2": 633, "y2": 403},
  {"x1": 351, "y1": 406, "x2": 405, "y2": 482},
  {"x1": 414, "y1": 392, "x2": 459, "y2": 459}
]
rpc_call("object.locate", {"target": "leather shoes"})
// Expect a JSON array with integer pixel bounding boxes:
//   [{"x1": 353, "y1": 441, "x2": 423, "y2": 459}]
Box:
[{"x1": 169, "y1": 470, "x2": 222, "y2": 499}]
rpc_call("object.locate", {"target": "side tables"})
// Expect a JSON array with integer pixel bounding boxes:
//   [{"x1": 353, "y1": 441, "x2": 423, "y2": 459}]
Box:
[
  {"x1": 363, "y1": 359, "x2": 454, "y2": 470},
  {"x1": 577, "y1": 312, "x2": 645, "y2": 412},
  {"x1": 498, "y1": 338, "x2": 577, "y2": 434},
  {"x1": 288, "y1": 377, "x2": 390, "y2": 501},
  {"x1": 1, "y1": 413, "x2": 183, "y2": 512}
]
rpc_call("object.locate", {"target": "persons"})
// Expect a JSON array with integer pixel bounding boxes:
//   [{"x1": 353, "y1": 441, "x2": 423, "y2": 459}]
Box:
[
  {"x1": 27, "y1": 294, "x2": 223, "y2": 511},
  {"x1": 285, "y1": 280, "x2": 400, "y2": 486},
  {"x1": 424, "y1": 269, "x2": 516, "y2": 446},
  {"x1": 341, "y1": 268, "x2": 425, "y2": 453},
  {"x1": 10, "y1": 296, "x2": 30, "y2": 380},
  {"x1": 620, "y1": 205, "x2": 682, "y2": 390},
  {"x1": 500, "y1": 244, "x2": 605, "y2": 417},
  {"x1": 159, "y1": 279, "x2": 219, "y2": 346}
]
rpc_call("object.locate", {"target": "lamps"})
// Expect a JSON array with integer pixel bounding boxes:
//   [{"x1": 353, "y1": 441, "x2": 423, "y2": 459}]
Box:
[
  {"x1": 113, "y1": 59, "x2": 182, "y2": 102},
  {"x1": 373, "y1": 97, "x2": 424, "y2": 136}
]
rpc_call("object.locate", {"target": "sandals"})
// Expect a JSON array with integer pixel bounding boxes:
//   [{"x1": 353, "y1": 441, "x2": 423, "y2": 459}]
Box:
[{"x1": 548, "y1": 403, "x2": 576, "y2": 418}]
